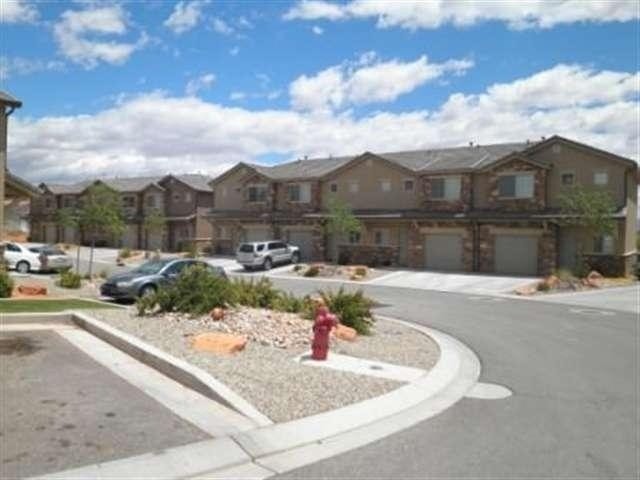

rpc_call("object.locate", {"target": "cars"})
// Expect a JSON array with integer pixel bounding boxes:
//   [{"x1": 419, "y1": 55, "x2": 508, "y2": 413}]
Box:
[
  {"x1": 98, "y1": 257, "x2": 230, "y2": 304},
  {"x1": 2, "y1": 241, "x2": 74, "y2": 273}
]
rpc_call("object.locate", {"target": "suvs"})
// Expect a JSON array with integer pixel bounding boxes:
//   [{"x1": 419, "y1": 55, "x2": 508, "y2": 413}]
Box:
[{"x1": 233, "y1": 239, "x2": 302, "y2": 270}]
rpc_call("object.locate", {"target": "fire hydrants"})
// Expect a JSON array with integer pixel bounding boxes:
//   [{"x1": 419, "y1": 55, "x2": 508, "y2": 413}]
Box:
[{"x1": 310, "y1": 305, "x2": 337, "y2": 359}]
